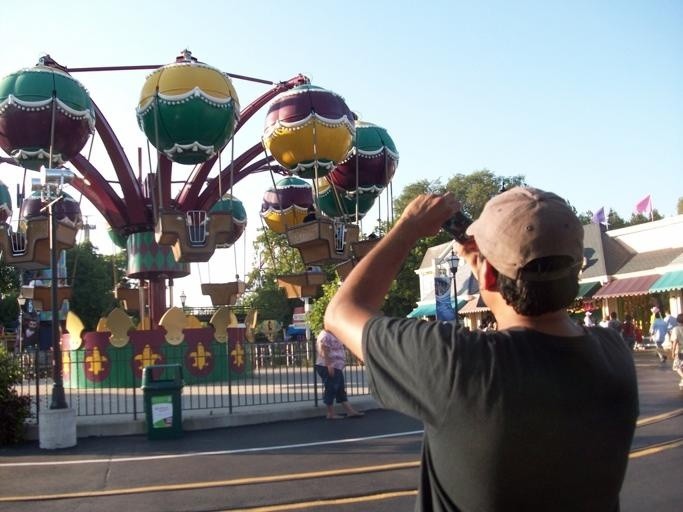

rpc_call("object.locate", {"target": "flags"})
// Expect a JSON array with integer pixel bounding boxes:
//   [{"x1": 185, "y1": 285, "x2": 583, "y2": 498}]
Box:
[
  {"x1": 591, "y1": 206, "x2": 605, "y2": 223},
  {"x1": 636, "y1": 194, "x2": 652, "y2": 213}
]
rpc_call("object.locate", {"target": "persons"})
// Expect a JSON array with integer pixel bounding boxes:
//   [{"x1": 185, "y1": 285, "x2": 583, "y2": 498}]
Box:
[
  {"x1": 314, "y1": 327, "x2": 366, "y2": 420},
  {"x1": 323, "y1": 185, "x2": 639, "y2": 512},
  {"x1": 24, "y1": 321, "x2": 38, "y2": 349},
  {"x1": 583, "y1": 306, "x2": 683, "y2": 388}
]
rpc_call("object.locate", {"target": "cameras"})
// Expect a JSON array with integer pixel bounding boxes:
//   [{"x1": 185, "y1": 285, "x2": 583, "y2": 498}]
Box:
[{"x1": 441, "y1": 209, "x2": 473, "y2": 244}]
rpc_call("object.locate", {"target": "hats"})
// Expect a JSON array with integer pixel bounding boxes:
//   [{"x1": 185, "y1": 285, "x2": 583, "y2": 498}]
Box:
[{"x1": 464, "y1": 184, "x2": 586, "y2": 284}]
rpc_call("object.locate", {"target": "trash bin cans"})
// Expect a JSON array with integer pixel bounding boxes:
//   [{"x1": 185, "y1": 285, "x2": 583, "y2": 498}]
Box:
[{"x1": 140, "y1": 363, "x2": 184, "y2": 441}]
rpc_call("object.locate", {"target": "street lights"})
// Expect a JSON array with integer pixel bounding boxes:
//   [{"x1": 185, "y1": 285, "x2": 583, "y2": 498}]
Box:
[
  {"x1": 180, "y1": 291, "x2": 185, "y2": 313},
  {"x1": 446, "y1": 247, "x2": 459, "y2": 327},
  {"x1": 30, "y1": 166, "x2": 75, "y2": 406},
  {"x1": 17, "y1": 294, "x2": 27, "y2": 396}
]
